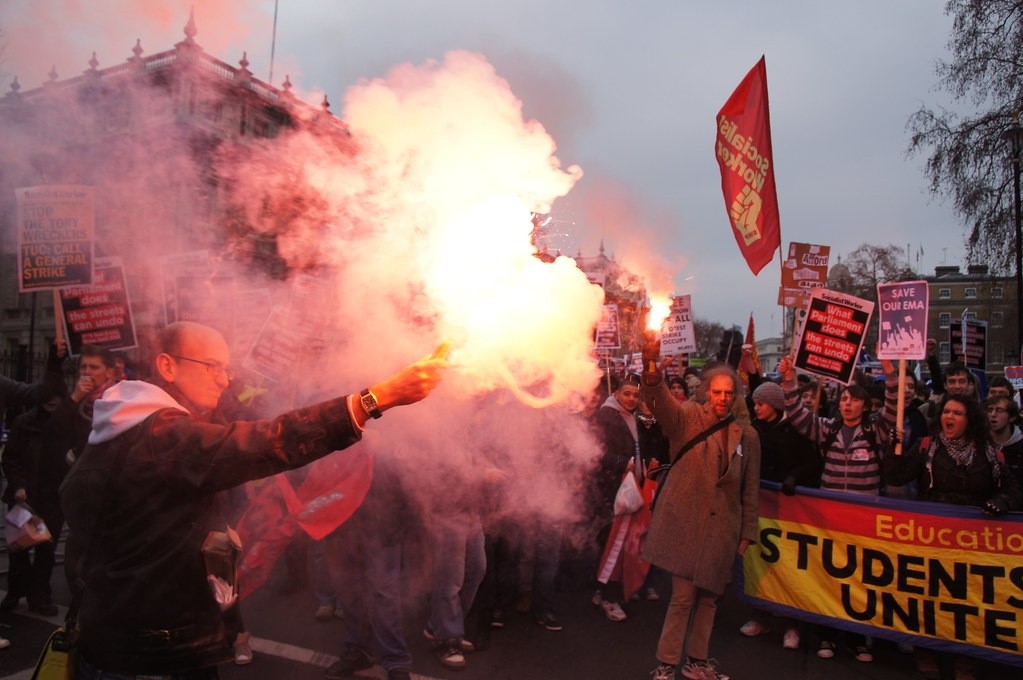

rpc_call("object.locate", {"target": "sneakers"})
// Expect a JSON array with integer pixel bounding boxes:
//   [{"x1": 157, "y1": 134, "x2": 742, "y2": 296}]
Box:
[
  {"x1": 848, "y1": 645, "x2": 874, "y2": 661},
  {"x1": 815, "y1": 639, "x2": 835, "y2": 658},
  {"x1": 431, "y1": 637, "x2": 466, "y2": 670},
  {"x1": 389, "y1": 670, "x2": 410, "y2": 680},
  {"x1": 782, "y1": 628, "x2": 800, "y2": 650},
  {"x1": 680, "y1": 656, "x2": 730, "y2": 680},
  {"x1": 649, "y1": 664, "x2": 676, "y2": 680},
  {"x1": 424, "y1": 627, "x2": 474, "y2": 652},
  {"x1": 740, "y1": 619, "x2": 771, "y2": 636},
  {"x1": 235, "y1": 632, "x2": 252, "y2": 665},
  {"x1": 324, "y1": 646, "x2": 374, "y2": 679}
]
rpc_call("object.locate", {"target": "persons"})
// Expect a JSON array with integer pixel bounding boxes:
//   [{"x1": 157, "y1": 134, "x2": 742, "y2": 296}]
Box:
[
  {"x1": 205, "y1": 358, "x2": 704, "y2": 680},
  {"x1": 742, "y1": 343, "x2": 1023, "y2": 678},
  {"x1": 641, "y1": 342, "x2": 761, "y2": 680},
  {"x1": 0, "y1": 338, "x2": 150, "y2": 648},
  {"x1": 61, "y1": 322, "x2": 456, "y2": 679}
]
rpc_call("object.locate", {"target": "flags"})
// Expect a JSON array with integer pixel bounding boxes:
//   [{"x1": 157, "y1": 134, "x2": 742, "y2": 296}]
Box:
[{"x1": 715, "y1": 56, "x2": 781, "y2": 276}]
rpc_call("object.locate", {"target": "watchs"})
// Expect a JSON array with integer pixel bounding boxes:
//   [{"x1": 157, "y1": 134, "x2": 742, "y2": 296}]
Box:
[{"x1": 360, "y1": 387, "x2": 383, "y2": 419}]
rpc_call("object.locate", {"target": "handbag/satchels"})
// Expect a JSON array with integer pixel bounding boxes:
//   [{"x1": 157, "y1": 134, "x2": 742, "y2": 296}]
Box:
[
  {"x1": 646, "y1": 465, "x2": 672, "y2": 511},
  {"x1": 29, "y1": 627, "x2": 80, "y2": 680},
  {"x1": 614, "y1": 457, "x2": 645, "y2": 515},
  {"x1": 4, "y1": 500, "x2": 53, "y2": 553}
]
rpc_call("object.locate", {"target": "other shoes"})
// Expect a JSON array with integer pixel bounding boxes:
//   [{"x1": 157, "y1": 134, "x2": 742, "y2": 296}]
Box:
[
  {"x1": 1, "y1": 591, "x2": 19, "y2": 612},
  {"x1": 914, "y1": 649, "x2": 939, "y2": 679},
  {"x1": 27, "y1": 593, "x2": 58, "y2": 615},
  {"x1": 315, "y1": 603, "x2": 344, "y2": 622},
  {"x1": 474, "y1": 586, "x2": 660, "y2": 649},
  {"x1": 952, "y1": 655, "x2": 976, "y2": 680},
  {"x1": 0, "y1": 639, "x2": 10, "y2": 649},
  {"x1": 864, "y1": 636, "x2": 872, "y2": 649},
  {"x1": 898, "y1": 643, "x2": 913, "y2": 654}
]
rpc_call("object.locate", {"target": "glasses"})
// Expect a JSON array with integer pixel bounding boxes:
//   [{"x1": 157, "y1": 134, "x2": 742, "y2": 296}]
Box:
[
  {"x1": 171, "y1": 354, "x2": 234, "y2": 381},
  {"x1": 987, "y1": 407, "x2": 1008, "y2": 413}
]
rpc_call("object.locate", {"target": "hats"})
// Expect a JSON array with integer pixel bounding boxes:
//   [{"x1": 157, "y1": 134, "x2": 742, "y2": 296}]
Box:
[{"x1": 752, "y1": 381, "x2": 784, "y2": 409}]
